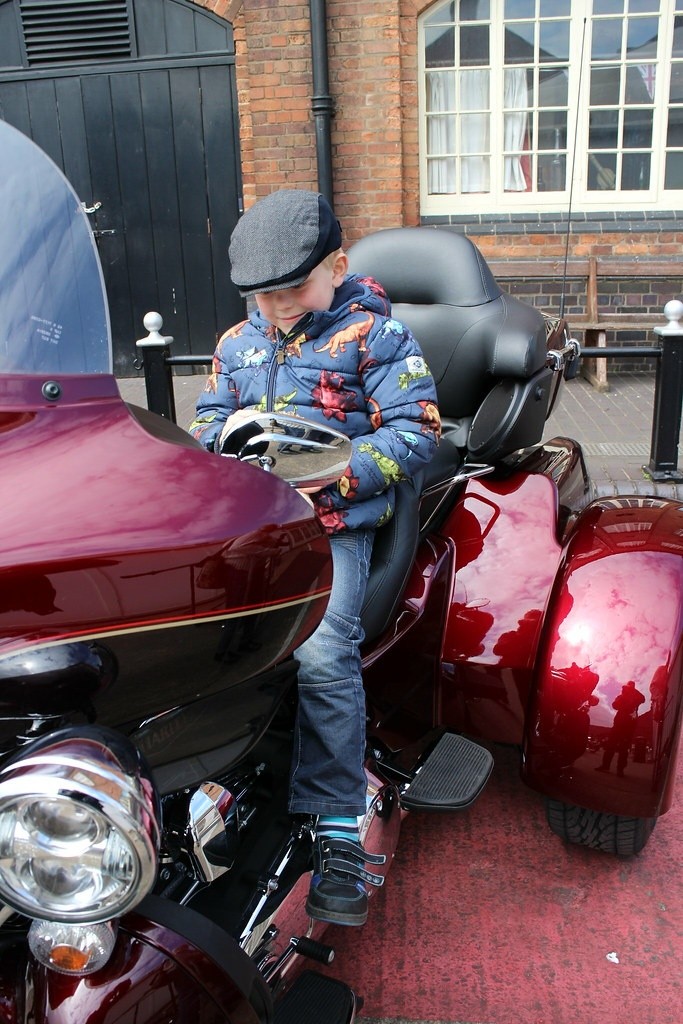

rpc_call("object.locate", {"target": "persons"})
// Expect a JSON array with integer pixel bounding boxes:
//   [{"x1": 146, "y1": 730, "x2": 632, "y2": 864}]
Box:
[{"x1": 188, "y1": 189, "x2": 441, "y2": 927}]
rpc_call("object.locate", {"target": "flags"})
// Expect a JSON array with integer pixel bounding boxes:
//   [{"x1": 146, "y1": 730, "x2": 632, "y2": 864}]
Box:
[{"x1": 638, "y1": 64, "x2": 656, "y2": 100}]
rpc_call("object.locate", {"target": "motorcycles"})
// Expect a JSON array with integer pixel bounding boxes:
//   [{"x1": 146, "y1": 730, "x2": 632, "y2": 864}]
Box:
[{"x1": 1, "y1": 120, "x2": 682, "y2": 1024}]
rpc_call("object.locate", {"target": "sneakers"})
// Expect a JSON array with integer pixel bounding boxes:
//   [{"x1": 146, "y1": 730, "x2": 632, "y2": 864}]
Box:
[{"x1": 304, "y1": 836, "x2": 387, "y2": 927}]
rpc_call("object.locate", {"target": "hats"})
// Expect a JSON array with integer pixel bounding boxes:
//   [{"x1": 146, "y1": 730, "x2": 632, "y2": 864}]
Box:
[{"x1": 229, "y1": 190, "x2": 343, "y2": 297}]
